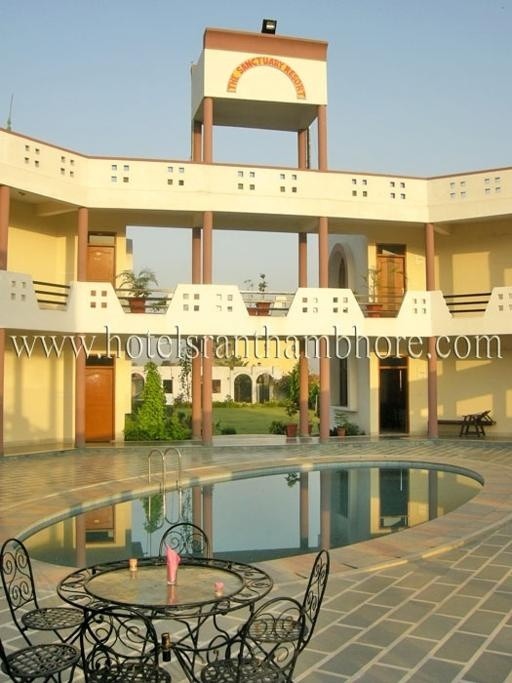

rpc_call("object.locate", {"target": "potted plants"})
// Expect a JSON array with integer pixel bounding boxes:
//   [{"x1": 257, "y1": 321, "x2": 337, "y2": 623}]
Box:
[
  {"x1": 332, "y1": 412, "x2": 350, "y2": 436},
  {"x1": 359, "y1": 261, "x2": 410, "y2": 317},
  {"x1": 115, "y1": 267, "x2": 161, "y2": 313},
  {"x1": 243, "y1": 273, "x2": 271, "y2": 315},
  {"x1": 284, "y1": 401, "x2": 302, "y2": 437}
]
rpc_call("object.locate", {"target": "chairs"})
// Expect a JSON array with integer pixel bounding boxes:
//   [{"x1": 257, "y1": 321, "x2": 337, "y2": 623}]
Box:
[{"x1": 459, "y1": 409, "x2": 491, "y2": 437}]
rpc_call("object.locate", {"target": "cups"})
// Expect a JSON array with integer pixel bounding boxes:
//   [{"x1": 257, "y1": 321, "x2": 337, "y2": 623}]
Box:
[
  {"x1": 166, "y1": 563, "x2": 178, "y2": 586},
  {"x1": 129, "y1": 558, "x2": 138, "y2": 572}
]
